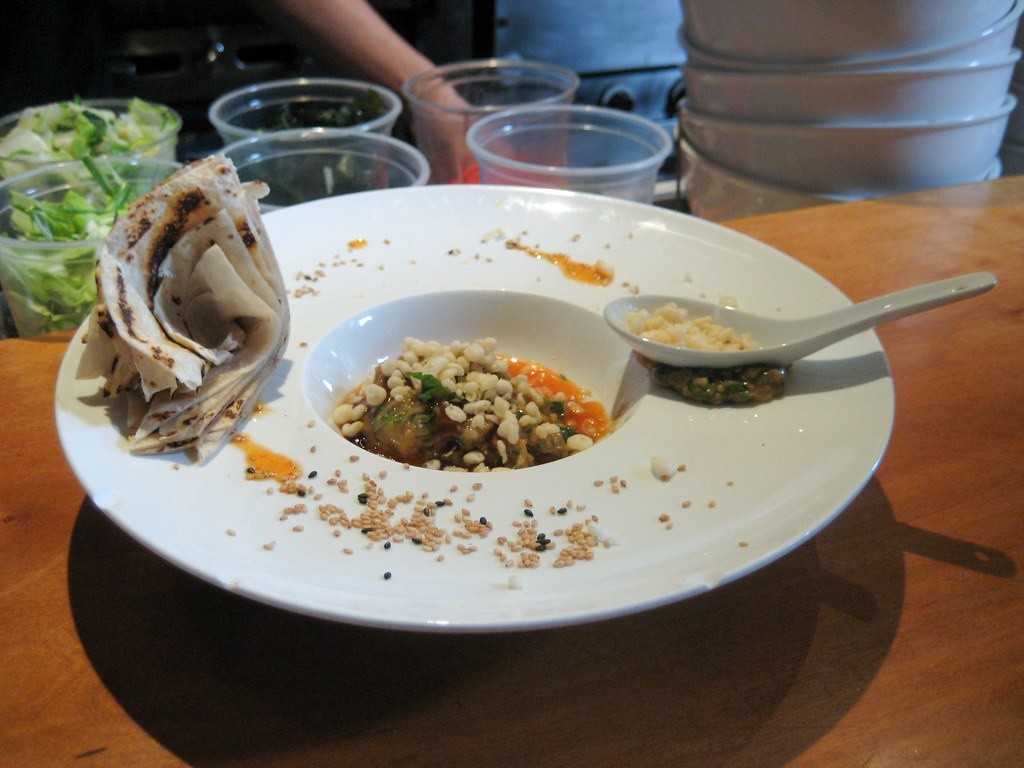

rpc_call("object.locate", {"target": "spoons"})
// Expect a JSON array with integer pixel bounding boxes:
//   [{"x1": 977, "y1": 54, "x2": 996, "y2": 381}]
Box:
[{"x1": 604, "y1": 272, "x2": 996, "y2": 367}]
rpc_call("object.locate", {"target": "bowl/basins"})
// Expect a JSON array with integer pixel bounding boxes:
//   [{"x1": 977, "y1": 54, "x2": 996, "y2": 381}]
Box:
[
  {"x1": 1, "y1": 101, "x2": 182, "y2": 194},
  {"x1": 0, "y1": 160, "x2": 186, "y2": 337},
  {"x1": 674, "y1": 0, "x2": 1022, "y2": 222},
  {"x1": 55, "y1": 185, "x2": 895, "y2": 634}
]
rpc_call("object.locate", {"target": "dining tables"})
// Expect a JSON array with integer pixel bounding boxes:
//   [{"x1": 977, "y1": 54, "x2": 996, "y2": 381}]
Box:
[{"x1": 2, "y1": 173, "x2": 1024, "y2": 768}]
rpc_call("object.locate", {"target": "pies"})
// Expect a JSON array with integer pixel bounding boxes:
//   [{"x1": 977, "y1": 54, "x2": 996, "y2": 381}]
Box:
[{"x1": 74, "y1": 149, "x2": 292, "y2": 468}]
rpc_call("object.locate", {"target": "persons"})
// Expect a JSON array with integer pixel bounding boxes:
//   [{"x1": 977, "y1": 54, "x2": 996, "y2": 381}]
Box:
[{"x1": 226, "y1": 0, "x2": 509, "y2": 184}]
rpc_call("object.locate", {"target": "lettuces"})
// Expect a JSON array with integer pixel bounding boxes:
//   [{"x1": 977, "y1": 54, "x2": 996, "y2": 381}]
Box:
[{"x1": 0, "y1": 92, "x2": 176, "y2": 337}]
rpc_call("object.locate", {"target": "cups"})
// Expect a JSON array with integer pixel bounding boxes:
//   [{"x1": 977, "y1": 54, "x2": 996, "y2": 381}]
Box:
[
  {"x1": 208, "y1": 78, "x2": 404, "y2": 189},
  {"x1": 465, "y1": 105, "x2": 672, "y2": 207},
  {"x1": 208, "y1": 127, "x2": 429, "y2": 216},
  {"x1": 405, "y1": 60, "x2": 580, "y2": 188}
]
